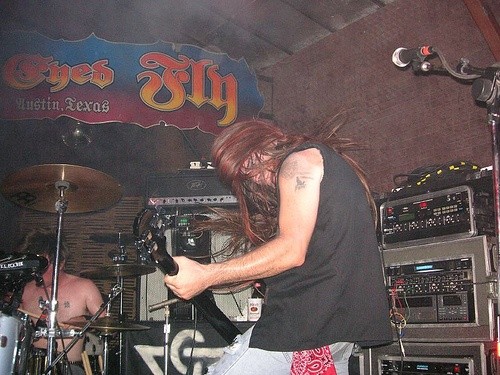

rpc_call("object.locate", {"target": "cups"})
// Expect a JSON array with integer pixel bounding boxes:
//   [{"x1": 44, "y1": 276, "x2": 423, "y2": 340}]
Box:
[{"x1": 248, "y1": 298, "x2": 262, "y2": 322}]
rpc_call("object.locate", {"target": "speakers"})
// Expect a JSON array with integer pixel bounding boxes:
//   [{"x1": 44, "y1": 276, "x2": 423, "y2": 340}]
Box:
[{"x1": 136, "y1": 210, "x2": 255, "y2": 322}]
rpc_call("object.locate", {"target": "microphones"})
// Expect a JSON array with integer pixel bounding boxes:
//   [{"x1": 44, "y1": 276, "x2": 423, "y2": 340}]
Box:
[
  {"x1": 37, "y1": 309, "x2": 47, "y2": 330},
  {"x1": 392, "y1": 46, "x2": 435, "y2": 67}
]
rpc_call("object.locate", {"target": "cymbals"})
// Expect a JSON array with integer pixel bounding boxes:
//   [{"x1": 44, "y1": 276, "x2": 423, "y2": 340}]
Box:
[
  {"x1": 61, "y1": 314, "x2": 152, "y2": 331},
  {"x1": 1, "y1": 163, "x2": 124, "y2": 215},
  {"x1": 80, "y1": 262, "x2": 157, "y2": 279},
  {"x1": 90, "y1": 230, "x2": 137, "y2": 244}
]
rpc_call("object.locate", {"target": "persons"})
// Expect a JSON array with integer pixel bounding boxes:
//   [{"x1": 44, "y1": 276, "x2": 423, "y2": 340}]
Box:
[
  {"x1": 3, "y1": 227, "x2": 106, "y2": 375},
  {"x1": 163, "y1": 105, "x2": 393, "y2": 375}
]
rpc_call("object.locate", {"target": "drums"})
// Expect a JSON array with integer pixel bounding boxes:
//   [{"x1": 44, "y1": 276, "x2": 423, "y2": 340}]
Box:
[{"x1": 0, "y1": 309, "x2": 34, "y2": 375}]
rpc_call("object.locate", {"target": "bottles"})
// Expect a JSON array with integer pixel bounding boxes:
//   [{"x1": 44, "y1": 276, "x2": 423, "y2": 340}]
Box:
[{"x1": 251, "y1": 283, "x2": 265, "y2": 304}]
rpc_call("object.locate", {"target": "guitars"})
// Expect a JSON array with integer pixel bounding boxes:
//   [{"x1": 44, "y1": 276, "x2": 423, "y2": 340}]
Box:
[{"x1": 131, "y1": 207, "x2": 241, "y2": 346}]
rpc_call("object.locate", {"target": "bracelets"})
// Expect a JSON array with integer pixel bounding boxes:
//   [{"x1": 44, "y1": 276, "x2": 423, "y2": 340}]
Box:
[
  {"x1": 82, "y1": 315, "x2": 97, "y2": 320},
  {"x1": 5, "y1": 294, "x2": 11, "y2": 298}
]
